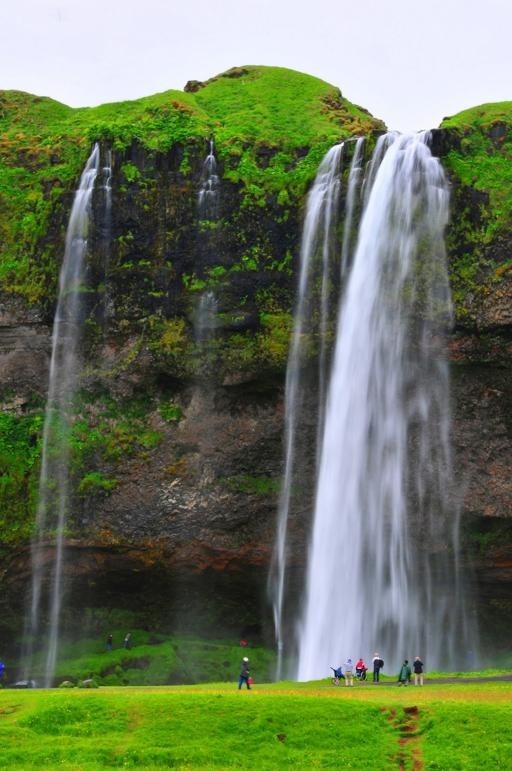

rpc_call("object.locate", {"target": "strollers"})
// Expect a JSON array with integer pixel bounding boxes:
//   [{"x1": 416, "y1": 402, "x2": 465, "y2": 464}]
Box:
[
  {"x1": 356, "y1": 665, "x2": 367, "y2": 680},
  {"x1": 331, "y1": 666, "x2": 345, "y2": 684}
]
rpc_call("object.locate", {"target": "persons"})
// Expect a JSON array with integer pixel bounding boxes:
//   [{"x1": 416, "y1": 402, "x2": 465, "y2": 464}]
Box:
[
  {"x1": 342, "y1": 658, "x2": 355, "y2": 686},
  {"x1": 105, "y1": 630, "x2": 113, "y2": 652},
  {"x1": 398, "y1": 661, "x2": 411, "y2": 687},
  {"x1": 371, "y1": 653, "x2": 384, "y2": 685},
  {"x1": 355, "y1": 659, "x2": 369, "y2": 682},
  {"x1": 328, "y1": 664, "x2": 346, "y2": 687},
  {"x1": 123, "y1": 631, "x2": 134, "y2": 650},
  {"x1": 0, "y1": 660, "x2": 7, "y2": 690},
  {"x1": 411, "y1": 656, "x2": 424, "y2": 688},
  {"x1": 237, "y1": 657, "x2": 252, "y2": 690}
]
